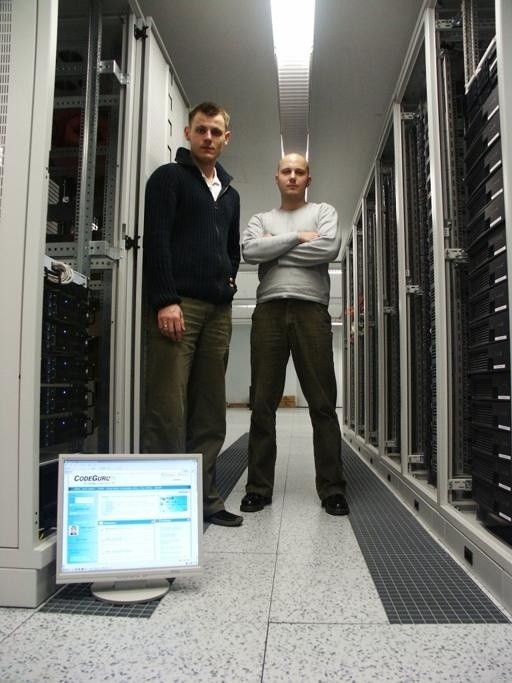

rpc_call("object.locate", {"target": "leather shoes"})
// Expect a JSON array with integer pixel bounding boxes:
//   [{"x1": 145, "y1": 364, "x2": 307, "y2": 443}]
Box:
[
  {"x1": 322, "y1": 494, "x2": 349, "y2": 514},
  {"x1": 240, "y1": 492, "x2": 272, "y2": 512}
]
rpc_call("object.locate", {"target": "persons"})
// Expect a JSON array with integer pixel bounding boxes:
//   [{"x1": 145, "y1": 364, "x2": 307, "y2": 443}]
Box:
[
  {"x1": 240, "y1": 154, "x2": 350, "y2": 515},
  {"x1": 140, "y1": 102, "x2": 243, "y2": 525}
]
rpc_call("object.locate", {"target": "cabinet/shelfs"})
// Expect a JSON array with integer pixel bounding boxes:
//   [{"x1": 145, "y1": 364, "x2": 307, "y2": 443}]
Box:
[
  {"x1": 0, "y1": 2, "x2": 144, "y2": 576},
  {"x1": 342, "y1": 2, "x2": 511, "y2": 603}
]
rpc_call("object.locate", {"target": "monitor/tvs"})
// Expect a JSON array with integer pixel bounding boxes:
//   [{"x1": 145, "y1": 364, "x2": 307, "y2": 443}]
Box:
[{"x1": 56, "y1": 453, "x2": 204, "y2": 605}]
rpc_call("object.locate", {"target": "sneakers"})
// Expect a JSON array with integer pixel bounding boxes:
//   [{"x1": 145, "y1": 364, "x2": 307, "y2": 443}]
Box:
[{"x1": 206, "y1": 510, "x2": 243, "y2": 526}]
